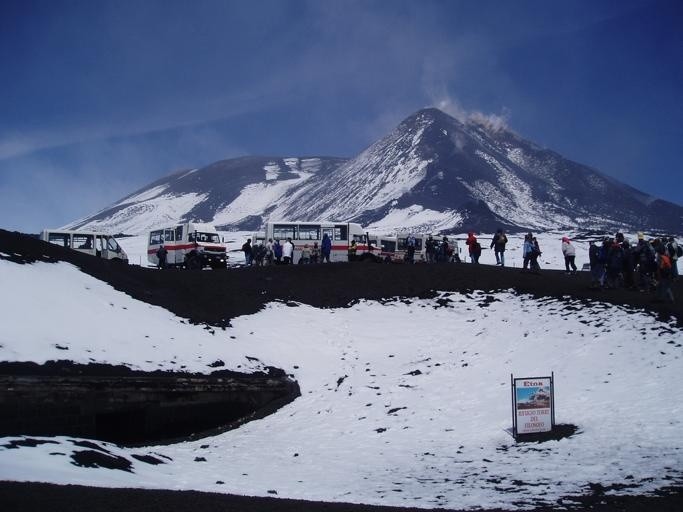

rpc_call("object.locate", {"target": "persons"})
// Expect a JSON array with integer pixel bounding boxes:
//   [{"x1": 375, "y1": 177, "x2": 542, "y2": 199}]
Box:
[
  {"x1": 562, "y1": 237, "x2": 577, "y2": 271},
  {"x1": 523, "y1": 232, "x2": 541, "y2": 271},
  {"x1": 470, "y1": 241, "x2": 481, "y2": 264},
  {"x1": 183, "y1": 250, "x2": 208, "y2": 270},
  {"x1": 302, "y1": 244, "x2": 311, "y2": 264},
  {"x1": 588, "y1": 232, "x2": 683, "y2": 302},
  {"x1": 466, "y1": 232, "x2": 476, "y2": 264},
  {"x1": 490, "y1": 228, "x2": 508, "y2": 266},
  {"x1": 320, "y1": 233, "x2": 331, "y2": 263},
  {"x1": 242, "y1": 238, "x2": 294, "y2": 264},
  {"x1": 189, "y1": 233, "x2": 195, "y2": 242},
  {"x1": 349, "y1": 234, "x2": 461, "y2": 264},
  {"x1": 156, "y1": 245, "x2": 168, "y2": 269},
  {"x1": 312, "y1": 244, "x2": 320, "y2": 264}
]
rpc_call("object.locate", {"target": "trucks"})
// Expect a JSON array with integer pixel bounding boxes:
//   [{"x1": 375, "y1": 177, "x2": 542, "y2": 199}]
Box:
[
  {"x1": 146, "y1": 222, "x2": 227, "y2": 268},
  {"x1": 253, "y1": 221, "x2": 458, "y2": 264},
  {"x1": 38, "y1": 229, "x2": 128, "y2": 264}
]
